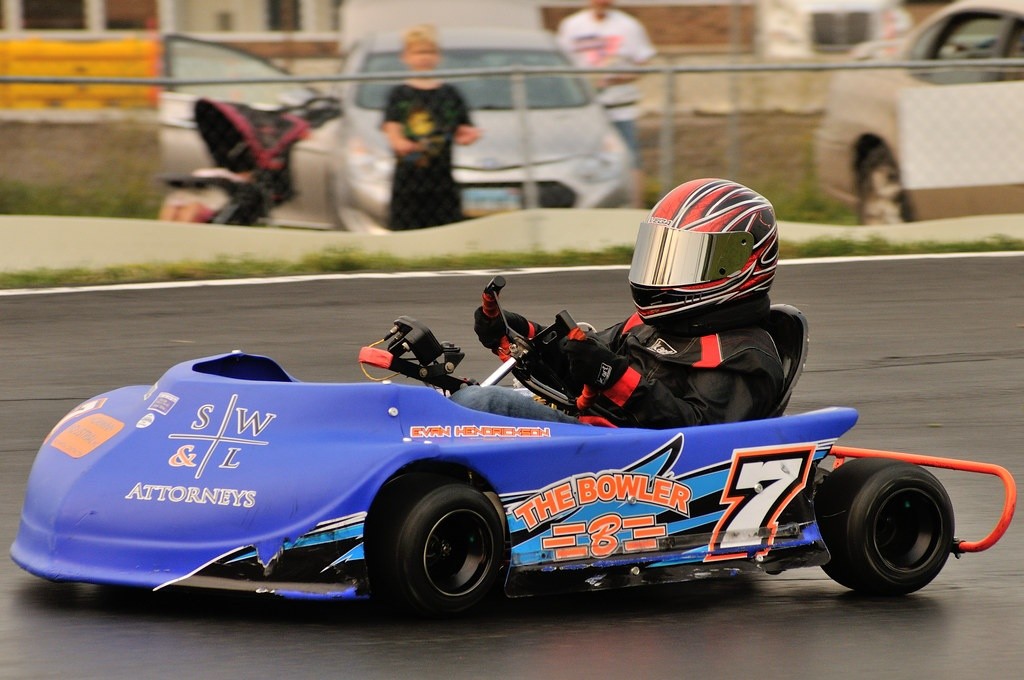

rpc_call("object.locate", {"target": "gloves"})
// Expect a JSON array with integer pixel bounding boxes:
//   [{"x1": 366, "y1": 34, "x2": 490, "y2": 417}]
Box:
[
  {"x1": 473, "y1": 306, "x2": 529, "y2": 349},
  {"x1": 559, "y1": 330, "x2": 642, "y2": 408}
]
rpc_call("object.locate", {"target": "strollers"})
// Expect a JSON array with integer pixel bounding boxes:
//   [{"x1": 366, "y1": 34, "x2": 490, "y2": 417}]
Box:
[{"x1": 156, "y1": 87, "x2": 349, "y2": 230}]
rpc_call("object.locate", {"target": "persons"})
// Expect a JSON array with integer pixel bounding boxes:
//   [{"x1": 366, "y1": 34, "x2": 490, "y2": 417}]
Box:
[
  {"x1": 449, "y1": 177, "x2": 785, "y2": 430},
  {"x1": 380, "y1": 22, "x2": 486, "y2": 232},
  {"x1": 553, "y1": 1, "x2": 657, "y2": 209}
]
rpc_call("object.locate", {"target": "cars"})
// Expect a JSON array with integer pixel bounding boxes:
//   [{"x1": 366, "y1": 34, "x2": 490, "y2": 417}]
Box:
[
  {"x1": 152, "y1": 17, "x2": 646, "y2": 230},
  {"x1": 812, "y1": 0, "x2": 1022, "y2": 225}
]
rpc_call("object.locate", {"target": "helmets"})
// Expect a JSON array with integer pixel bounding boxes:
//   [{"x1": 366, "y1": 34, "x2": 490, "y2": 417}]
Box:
[{"x1": 627, "y1": 177, "x2": 780, "y2": 326}]
